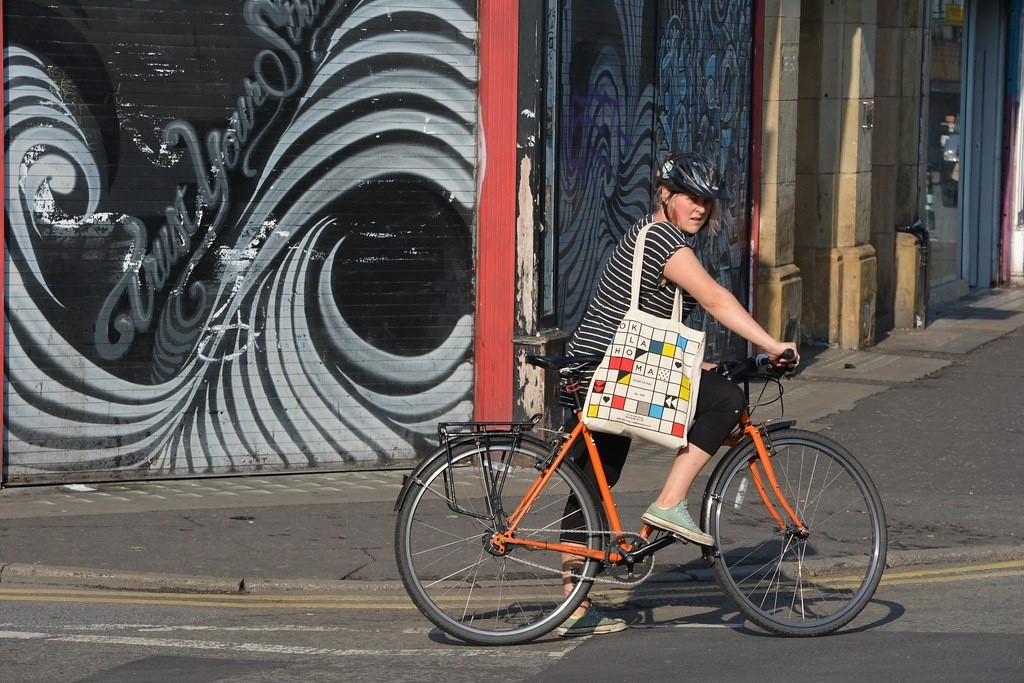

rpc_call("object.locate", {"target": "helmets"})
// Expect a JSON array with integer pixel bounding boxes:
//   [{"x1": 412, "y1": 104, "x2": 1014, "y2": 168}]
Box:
[{"x1": 655, "y1": 153, "x2": 723, "y2": 198}]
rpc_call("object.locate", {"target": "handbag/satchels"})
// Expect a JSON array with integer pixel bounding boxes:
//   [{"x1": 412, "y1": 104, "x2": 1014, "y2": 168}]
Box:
[{"x1": 581, "y1": 221, "x2": 707, "y2": 450}]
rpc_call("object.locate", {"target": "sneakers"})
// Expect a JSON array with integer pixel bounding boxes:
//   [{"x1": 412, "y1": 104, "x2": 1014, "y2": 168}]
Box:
[
  {"x1": 556, "y1": 604, "x2": 627, "y2": 636},
  {"x1": 640, "y1": 499, "x2": 715, "y2": 547}
]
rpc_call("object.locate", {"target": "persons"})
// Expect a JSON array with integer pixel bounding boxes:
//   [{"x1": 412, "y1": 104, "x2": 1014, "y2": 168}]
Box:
[{"x1": 559, "y1": 152, "x2": 800, "y2": 640}]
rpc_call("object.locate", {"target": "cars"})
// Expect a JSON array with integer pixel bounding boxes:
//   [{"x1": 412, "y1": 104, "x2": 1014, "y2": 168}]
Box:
[{"x1": 941, "y1": 109, "x2": 960, "y2": 197}]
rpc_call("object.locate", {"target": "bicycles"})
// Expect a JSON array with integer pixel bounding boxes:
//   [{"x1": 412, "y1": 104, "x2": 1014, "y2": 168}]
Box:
[{"x1": 393, "y1": 348, "x2": 889, "y2": 646}]
